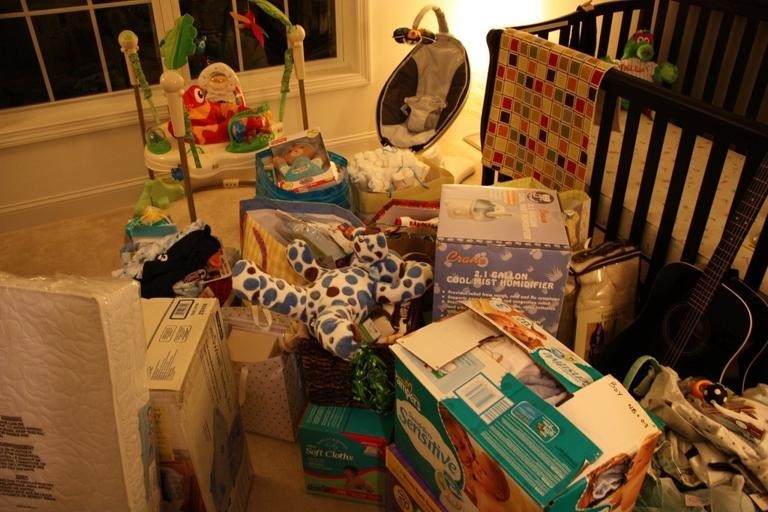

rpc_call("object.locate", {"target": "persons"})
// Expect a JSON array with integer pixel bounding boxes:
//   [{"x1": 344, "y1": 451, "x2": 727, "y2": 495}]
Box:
[
  {"x1": 439, "y1": 406, "x2": 516, "y2": 512},
  {"x1": 344, "y1": 464, "x2": 373, "y2": 493}
]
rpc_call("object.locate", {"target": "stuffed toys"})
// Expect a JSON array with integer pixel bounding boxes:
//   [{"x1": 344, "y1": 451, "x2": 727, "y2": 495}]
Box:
[
  {"x1": 600, "y1": 29, "x2": 678, "y2": 118},
  {"x1": 231, "y1": 226, "x2": 434, "y2": 361}
]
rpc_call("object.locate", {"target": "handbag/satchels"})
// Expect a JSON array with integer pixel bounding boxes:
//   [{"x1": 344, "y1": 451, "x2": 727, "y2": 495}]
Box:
[
  {"x1": 214, "y1": 305, "x2": 307, "y2": 443},
  {"x1": 239, "y1": 148, "x2": 450, "y2": 310}
]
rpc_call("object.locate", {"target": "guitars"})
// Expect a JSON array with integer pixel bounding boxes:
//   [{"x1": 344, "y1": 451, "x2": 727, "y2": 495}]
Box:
[{"x1": 594, "y1": 160, "x2": 768, "y2": 396}]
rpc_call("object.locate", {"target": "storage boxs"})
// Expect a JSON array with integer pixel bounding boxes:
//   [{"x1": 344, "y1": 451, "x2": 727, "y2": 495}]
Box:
[
  {"x1": 380, "y1": 297, "x2": 660, "y2": 511},
  {"x1": 294, "y1": 306, "x2": 421, "y2": 506},
  {"x1": 143, "y1": 297, "x2": 256, "y2": 511},
  {"x1": 434, "y1": 182, "x2": 571, "y2": 338}
]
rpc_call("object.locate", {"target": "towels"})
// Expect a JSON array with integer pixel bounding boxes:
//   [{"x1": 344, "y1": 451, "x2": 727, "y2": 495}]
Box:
[{"x1": 481, "y1": 27, "x2": 622, "y2": 193}]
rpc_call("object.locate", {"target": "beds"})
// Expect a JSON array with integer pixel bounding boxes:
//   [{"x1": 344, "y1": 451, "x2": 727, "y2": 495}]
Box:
[{"x1": 478, "y1": 0, "x2": 767, "y2": 328}]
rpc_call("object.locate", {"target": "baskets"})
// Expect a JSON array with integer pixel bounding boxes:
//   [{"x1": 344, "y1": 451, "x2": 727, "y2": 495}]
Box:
[{"x1": 296, "y1": 275, "x2": 423, "y2": 411}]
